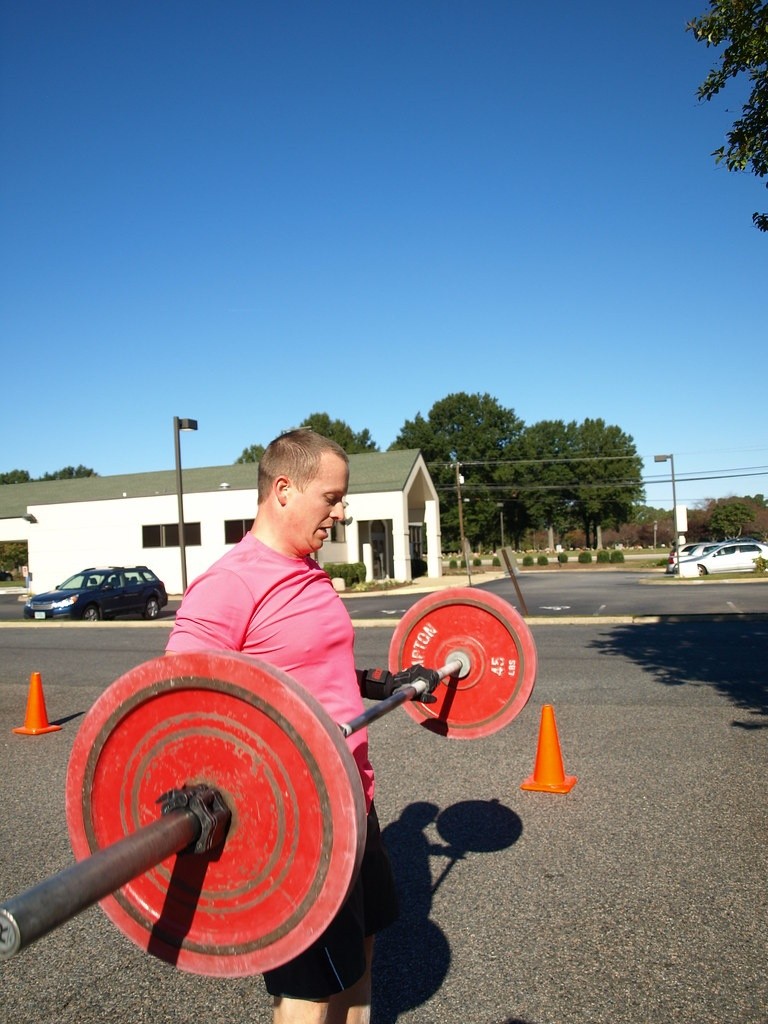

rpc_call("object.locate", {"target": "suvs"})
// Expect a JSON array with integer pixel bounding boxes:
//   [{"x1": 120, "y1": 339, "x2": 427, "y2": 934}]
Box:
[{"x1": 24, "y1": 565, "x2": 168, "y2": 626}]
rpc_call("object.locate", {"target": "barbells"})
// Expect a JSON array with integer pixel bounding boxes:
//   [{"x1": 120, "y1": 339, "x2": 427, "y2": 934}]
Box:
[{"x1": 0, "y1": 586, "x2": 537, "y2": 980}]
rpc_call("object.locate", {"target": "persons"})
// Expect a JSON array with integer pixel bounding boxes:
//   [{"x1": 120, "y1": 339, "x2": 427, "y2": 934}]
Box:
[{"x1": 163, "y1": 429, "x2": 440, "y2": 1024}]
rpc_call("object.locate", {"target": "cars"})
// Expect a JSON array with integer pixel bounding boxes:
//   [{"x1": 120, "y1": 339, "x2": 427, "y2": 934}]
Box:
[
  {"x1": 673, "y1": 539, "x2": 768, "y2": 577},
  {"x1": 0, "y1": 571, "x2": 13, "y2": 582},
  {"x1": 667, "y1": 542, "x2": 725, "y2": 575}
]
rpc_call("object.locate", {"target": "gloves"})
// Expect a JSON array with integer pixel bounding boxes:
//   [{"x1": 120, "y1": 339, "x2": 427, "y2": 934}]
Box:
[{"x1": 392, "y1": 664, "x2": 440, "y2": 704}]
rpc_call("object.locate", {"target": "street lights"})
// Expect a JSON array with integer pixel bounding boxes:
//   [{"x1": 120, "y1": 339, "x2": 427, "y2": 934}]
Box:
[
  {"x1": 173, "y1": 415, "x2": 201, "y2": 596},
  {"x1": 655, "y1": 454, "x2": 680, "y2": 579}
]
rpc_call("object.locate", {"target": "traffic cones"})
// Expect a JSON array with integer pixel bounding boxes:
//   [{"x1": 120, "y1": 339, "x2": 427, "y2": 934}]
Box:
[
  {"x1": 13, "y1": 673, "x2": 62, "y2": 735},
  {"x1": 523, "y1": 704, "x2": 591, "y2": 798}
]
